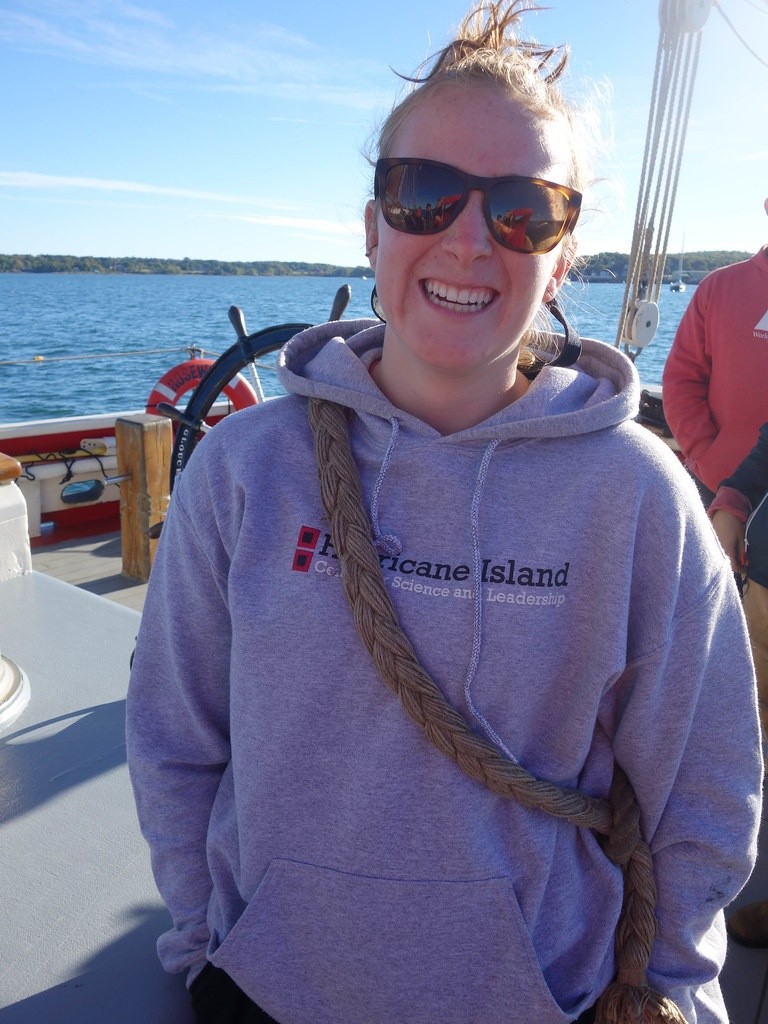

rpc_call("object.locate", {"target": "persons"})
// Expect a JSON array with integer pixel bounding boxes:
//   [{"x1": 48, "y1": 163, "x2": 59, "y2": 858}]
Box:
[
  {"x1": 660, "y1": 199, "x2": 768, "y2": 950},
  {"x1": 125, "y1": 0, "x2": 764, "y2": 1024}
]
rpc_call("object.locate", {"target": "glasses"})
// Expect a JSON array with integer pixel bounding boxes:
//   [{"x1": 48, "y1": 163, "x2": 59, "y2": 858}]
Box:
[{"x1": 374, "y1": 158, "x2": 583, "y2": 255}]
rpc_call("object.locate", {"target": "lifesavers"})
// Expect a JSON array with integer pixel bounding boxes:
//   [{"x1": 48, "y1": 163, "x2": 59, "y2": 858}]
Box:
[{"x1": 141, "y1": 358, "x2": 260, "y2": 455}]
